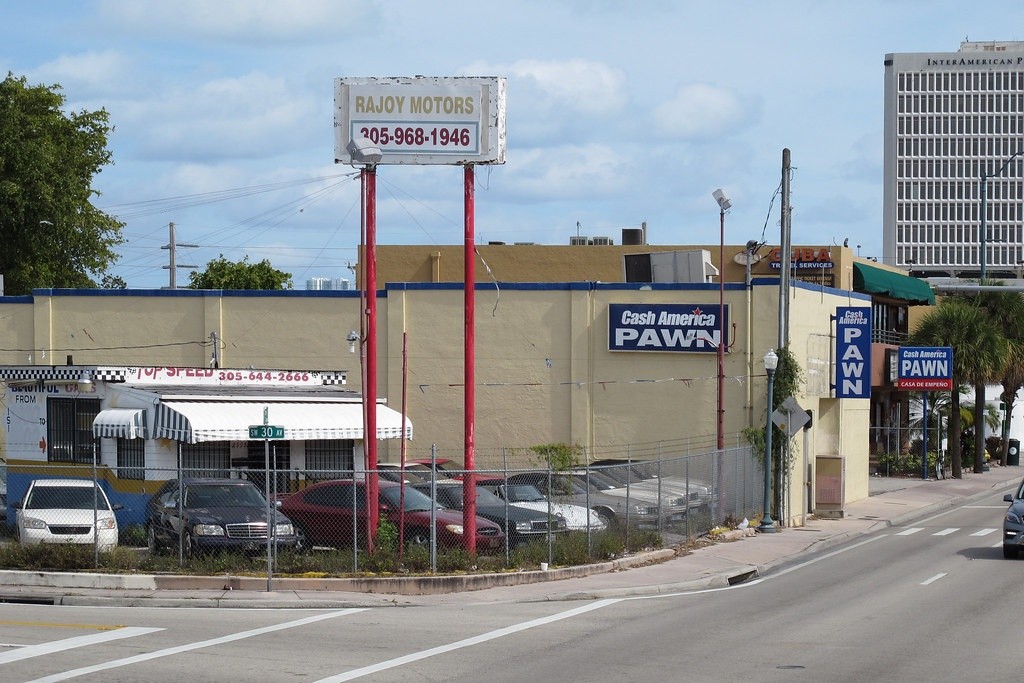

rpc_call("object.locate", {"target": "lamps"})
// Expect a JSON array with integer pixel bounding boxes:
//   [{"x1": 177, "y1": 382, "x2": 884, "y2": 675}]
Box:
[{"x1": 77, "y1": 371, "x2": 92, "y2": 391}]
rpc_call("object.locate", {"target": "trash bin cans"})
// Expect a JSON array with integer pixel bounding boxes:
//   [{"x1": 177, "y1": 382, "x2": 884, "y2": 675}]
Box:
[{"x1": 1006, "y1": 439, "x2": 1020, "y2": 465}]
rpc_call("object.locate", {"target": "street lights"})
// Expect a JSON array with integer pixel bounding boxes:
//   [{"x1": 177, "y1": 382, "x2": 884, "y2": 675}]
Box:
[
  {"x1": 758, "y1": 348, "x2": 779, "y2": 532},
  {"x1": 712, "y1": 188, "x2": 731, "y2": 527},
  {"x1": 347, "y1": 138, "x2": 382, "y2": 552}
]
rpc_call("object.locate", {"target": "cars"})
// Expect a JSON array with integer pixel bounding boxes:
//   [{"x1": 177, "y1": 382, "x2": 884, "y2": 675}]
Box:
[
  {"x1": 376, "y1": 462, "x2": 463, "y2": 485},
  {"x1": 406, "y1": 459, "x2": 505, "y2": 482},
  {"x1": 10, "y1": 480, "x2": 124, "y2": 552},
  {"x1": 270, "y1": 479, "x2": 506, "y2": 556},
  {"x1": 559, "y1": 459, "x2": 719, "y2": 512},
  {"x1": 412, "y1": 484, "x2": 571, "y2": 550},
  {"x1": 1003, "y1": 477, "x2": 1024, "y2": 560},
  {"x1": 508, "y1": 473, "x2": 664, "y2": 534},
  {"x1": 550, "y1": 471, "x2": 687, "y2": 523},
  {"x1": 145, "y1": 478, "x2": 298, "y2": 560},
  {"x1": 476, "y1": 480, "x2": 608, "y2": 533}
]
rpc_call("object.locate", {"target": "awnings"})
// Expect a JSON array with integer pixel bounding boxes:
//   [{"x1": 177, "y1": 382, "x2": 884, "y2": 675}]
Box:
[
  {"x1": 706, "y1": 262, "x2": 719, "y2": 276},
  {"x1": 92, "y1": 407, "x2": 149, "y2": 440},
  {"x1": 152, "y1": 402, "x2": 412, "y2": 443},
  {"x1": 853, "y1": 261, "x2": 936, "y2": 306}
]
